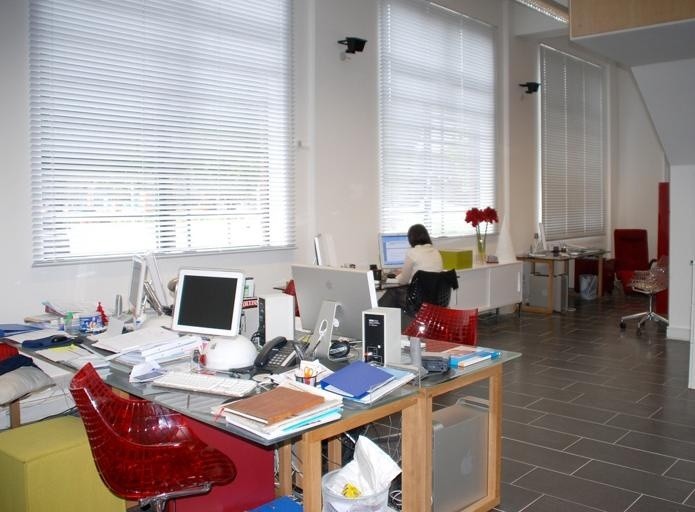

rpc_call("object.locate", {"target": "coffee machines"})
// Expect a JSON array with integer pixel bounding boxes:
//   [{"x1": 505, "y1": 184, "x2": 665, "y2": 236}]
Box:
[{"x1": 303, "y1": 367, "x2": 313, "y2": 377}]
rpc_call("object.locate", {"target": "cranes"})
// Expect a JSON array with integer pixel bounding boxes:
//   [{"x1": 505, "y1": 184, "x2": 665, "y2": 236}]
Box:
[
  {"x1": 320, "y1": 360, "x2": 395, "y2": 399},
  {"x1": 0, "y1": 323, "x2": 42, "y2": 337}
]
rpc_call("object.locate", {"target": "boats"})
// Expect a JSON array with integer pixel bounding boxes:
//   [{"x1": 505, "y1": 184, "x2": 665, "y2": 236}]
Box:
[
  {"x1": 258, "y1": 293, "x2": 294, "y2": 348},
  {"x1": 361, "y1": 306, "x2": 401, "y2": 367}
]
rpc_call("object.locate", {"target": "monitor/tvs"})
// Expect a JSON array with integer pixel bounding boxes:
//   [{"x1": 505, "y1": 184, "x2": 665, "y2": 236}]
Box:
[
  {"x1": 441, "y1": 345, "x2": 501, "y2": 367},
  {"x1": 222, "y1": 358, "x2": 415, "y2": 444},
  {"x1": 5, "y1": 301, "x2": 206, "y2": 377}
]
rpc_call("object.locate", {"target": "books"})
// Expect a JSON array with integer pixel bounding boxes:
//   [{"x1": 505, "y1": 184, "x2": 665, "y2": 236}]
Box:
[{"x1": 438, "y1": 249, "x2": 473, "y2": 270}]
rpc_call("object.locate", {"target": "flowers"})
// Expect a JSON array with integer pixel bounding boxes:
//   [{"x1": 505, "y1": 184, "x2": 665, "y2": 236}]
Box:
[{"x1": 464, "y1": 207, "x2": 499, "y2": 252}]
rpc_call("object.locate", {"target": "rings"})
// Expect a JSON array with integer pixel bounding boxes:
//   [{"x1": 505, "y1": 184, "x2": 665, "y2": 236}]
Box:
[{"x1": 293, "y1": 340, "x2": 321, "y2": 360}]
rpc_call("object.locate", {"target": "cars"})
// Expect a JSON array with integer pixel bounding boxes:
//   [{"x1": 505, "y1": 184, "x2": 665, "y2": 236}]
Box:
[
  {"x1": 294, "y1": 359, "x2": 320, "y2": 387},
  {"x1": 373, "y1": 270, "x2": 381, "y2": 280},
  {"x1": 191, "y1": 350, "x2": 207, "y2": 374}
]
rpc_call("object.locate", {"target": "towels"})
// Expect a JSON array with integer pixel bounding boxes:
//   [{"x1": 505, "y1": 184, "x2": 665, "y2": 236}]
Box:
[
  {"x1": 579, "y1": 274, "x2": 598, "y2": 301},
  {"x1": 321, "y1": 468, "x2": 392, "y2": 512}
]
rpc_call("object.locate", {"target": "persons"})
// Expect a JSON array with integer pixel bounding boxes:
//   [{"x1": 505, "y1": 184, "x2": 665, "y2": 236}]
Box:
[{"x1": 378, "y1": 223, "x2": 445, "y2": 314}]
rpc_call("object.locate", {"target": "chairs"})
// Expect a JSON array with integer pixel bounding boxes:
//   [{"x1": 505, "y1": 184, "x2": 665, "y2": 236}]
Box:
[
  {"x1": 406, "y1": 269, "x2": 452, "y2": 317},
  {"x1": 614, "y1": 229, "x2": 669, "y2": 337}
]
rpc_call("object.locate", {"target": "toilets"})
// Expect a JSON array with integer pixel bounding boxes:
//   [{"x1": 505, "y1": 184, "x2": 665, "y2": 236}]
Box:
[{"x1": 254, "y1": 336, "x2": 310, "y2": 374}]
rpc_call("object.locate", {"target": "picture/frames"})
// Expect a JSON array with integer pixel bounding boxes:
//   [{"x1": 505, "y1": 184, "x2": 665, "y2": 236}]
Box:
[{"x1": 516, "y1": 248, "x2": 611, "y2": 314}]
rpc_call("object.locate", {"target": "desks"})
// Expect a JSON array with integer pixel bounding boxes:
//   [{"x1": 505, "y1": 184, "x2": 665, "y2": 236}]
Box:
[
  {"x1": 516, "y1": 248, "x2": 611, "y2": 314},
  {"x1": 0, "y1": 308, "x2": 523, "y2": 512}
]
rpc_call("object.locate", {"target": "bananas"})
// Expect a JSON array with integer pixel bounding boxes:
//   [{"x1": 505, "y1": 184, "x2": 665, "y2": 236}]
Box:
[{"x1": 151, "y1": 371, "x2": 257, "y2": 397}]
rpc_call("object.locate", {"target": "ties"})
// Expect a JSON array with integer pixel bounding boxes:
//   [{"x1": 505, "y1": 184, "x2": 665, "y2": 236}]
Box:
[
  {"x1": 126, "y1": 256, "x2": 163, "y2": 318},
  {"x1": 378, "y1": 232, "x2": 412, "y2": 268},
  {"x1": 291, "y1": 265, "x2": 378, "y2": 362},
  {"x1": 145, "y1": 253, "x2": 168, "y2": 310},
  {"x1": 172, "y1": 269, "x2": 244, "y2": 337},
  {"x1": 314, "y1": 233, "x2": 331, "y2": 265}
]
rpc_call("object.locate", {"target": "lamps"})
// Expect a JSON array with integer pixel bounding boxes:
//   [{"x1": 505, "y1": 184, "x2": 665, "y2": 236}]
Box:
[
  {"x1": 614, "y1": 229, "x2": 669, "y2": 337},
  {"x1": 406, "y1": 269, "x2": 452, "y2": 317}
]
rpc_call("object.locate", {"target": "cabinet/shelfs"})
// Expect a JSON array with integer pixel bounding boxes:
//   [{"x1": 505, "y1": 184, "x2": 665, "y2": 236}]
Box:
[{"x1": 115, "y1": 295, "x2": 122, "y2": 316}]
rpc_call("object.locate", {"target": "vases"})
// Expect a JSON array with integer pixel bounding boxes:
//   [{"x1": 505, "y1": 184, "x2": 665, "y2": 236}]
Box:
[{"x1": 464, "y1": 207, "x2": 499, "y2": 252}]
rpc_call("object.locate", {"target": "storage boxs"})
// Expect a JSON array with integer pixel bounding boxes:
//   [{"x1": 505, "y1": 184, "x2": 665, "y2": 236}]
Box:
[{"x1": 438, "y1": 249, "x2": 473, "y2": 270}]
